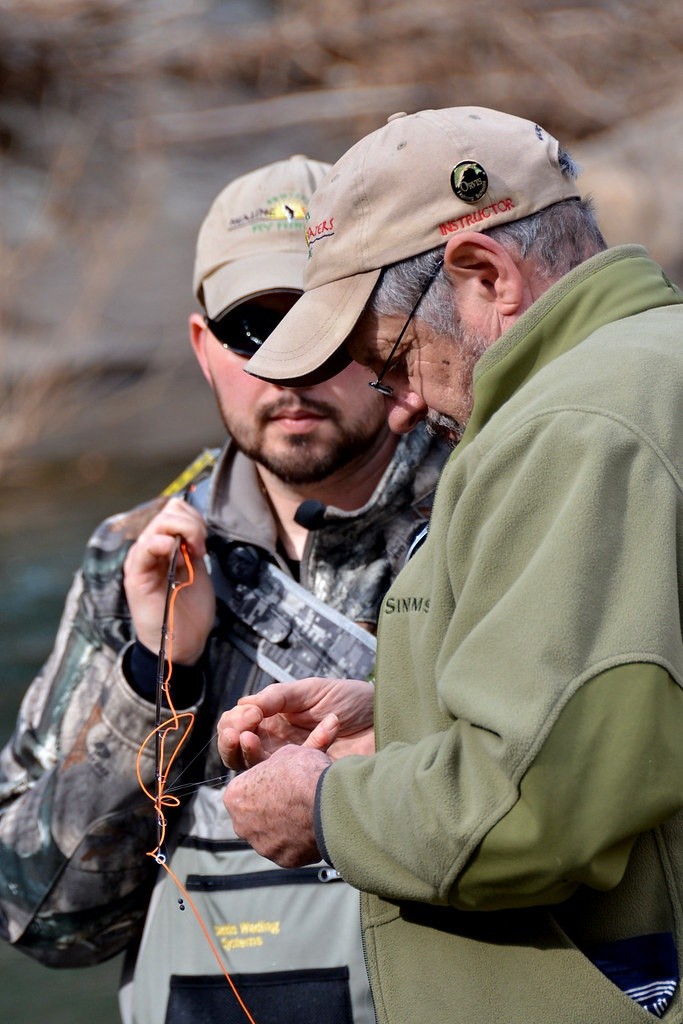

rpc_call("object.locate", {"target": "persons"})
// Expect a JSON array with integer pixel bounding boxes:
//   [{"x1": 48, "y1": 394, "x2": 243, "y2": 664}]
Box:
[
  {"x1": 243, "y1": 106, "x2": 683, "y2": 1023},
  {"x1": 2, "y1": 151, "x2": 452, "y2": 1023}
]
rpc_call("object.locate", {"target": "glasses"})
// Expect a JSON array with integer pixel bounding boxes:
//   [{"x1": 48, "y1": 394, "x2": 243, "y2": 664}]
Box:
[
  {"x1": 367, "y1": 256, "x2": 445, "y2": 397},
  {"x1": 204, "y1": 301, "x2": 300, "y2": 356}
]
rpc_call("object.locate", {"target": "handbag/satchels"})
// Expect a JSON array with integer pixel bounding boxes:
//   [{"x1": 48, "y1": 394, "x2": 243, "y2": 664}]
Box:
[{"x1": 117, "y1": 783, "x2": 375, "y2": 1024}]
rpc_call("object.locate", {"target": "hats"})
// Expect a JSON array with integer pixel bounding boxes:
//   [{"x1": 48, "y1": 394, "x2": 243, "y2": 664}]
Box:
[
  {"x1": 192, "y1": 154, "x2": 333, "y2": 322},
  {"x1": 243, "y1": 107, "x2": 583, "y2": 389}
]
